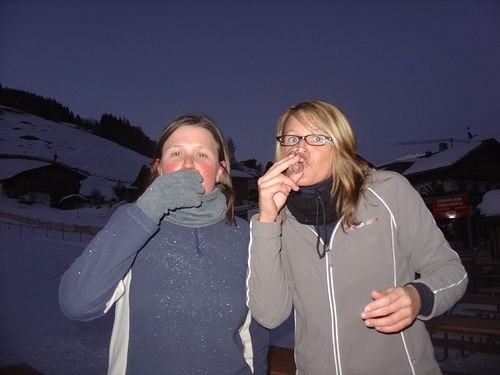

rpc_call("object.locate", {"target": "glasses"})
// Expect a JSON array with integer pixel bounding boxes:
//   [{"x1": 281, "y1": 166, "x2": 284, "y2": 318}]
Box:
[{"x1": 276, "y1": 133, "x2": 333, "y2": 147}]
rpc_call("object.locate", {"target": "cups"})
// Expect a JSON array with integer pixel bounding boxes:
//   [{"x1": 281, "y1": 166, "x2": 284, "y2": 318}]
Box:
[{"x1": 287, "y1": 146, "x2": 310, "y2": 174}]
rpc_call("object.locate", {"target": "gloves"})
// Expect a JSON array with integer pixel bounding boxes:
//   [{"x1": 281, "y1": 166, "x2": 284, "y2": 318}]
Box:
[{"x1": 137, "y1": 169, "x2": 206, "y2": 225}]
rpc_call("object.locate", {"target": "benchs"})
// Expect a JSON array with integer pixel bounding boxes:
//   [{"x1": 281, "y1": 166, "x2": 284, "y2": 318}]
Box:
[{"x1": 268, "y1": 286, "x2": 500, "y2": 375}]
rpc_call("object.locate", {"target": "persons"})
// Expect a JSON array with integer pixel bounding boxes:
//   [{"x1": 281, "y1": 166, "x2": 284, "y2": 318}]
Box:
[
  {"x1": 245, "y1": 98, "x2": 468, "y2": 375},
  {"x1": 59, "y1": 115, "x2": 296, "y2": 375}
]
